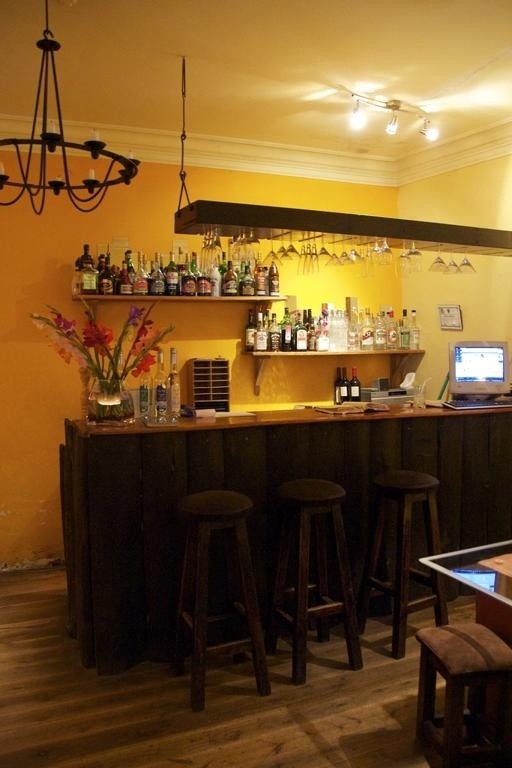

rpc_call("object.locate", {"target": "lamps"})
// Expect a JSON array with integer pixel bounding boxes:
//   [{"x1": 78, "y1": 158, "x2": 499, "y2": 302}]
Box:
[
  {"x1": 386, "y1": 116, "x2": 398, "y2": 134},
  {"x1": 0, "y1": 1, "x2": 140, "y2": 213},
  {"x1": 420, "y1": 129, "x2": 438, "y2": 141}
]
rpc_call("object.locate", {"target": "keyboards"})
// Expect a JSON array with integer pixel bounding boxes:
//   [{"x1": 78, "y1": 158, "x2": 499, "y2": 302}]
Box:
[{"x1": 441, "y1": 399, "x2": 512, "y2": 409}]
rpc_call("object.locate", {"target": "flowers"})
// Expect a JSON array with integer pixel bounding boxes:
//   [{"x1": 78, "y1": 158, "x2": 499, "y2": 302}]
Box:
[{"x1": 29, "y1": 296, "x2": 176, "y2": 420}]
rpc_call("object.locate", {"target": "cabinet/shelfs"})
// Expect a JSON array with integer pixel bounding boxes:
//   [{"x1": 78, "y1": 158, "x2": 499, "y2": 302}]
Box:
[{"x1": 74, "y1": 293, "x2": 425, "y2": 396}]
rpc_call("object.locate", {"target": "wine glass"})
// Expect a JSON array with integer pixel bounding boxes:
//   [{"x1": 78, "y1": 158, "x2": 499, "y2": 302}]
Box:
[
  {"x1": 427, "y1": 252, "x2": 450, "y2": 273},
  {"x1": 444, "y1": 253, "x2": 462, "y2": 274},
  {"x1": 198, "y1": 226, "x2": 263, "y2": 272},
  {"x1": 456, "y1": 253, "x2": 476, "y2": 274},
  {"x1": 315, "y1": 233, "x2": 424, "y2": 279},
  {"x1": 263, "y1": 230, "x2": 319, "y2": 276}
]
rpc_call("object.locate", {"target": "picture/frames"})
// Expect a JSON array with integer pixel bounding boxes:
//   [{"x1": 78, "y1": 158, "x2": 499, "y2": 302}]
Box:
[{"x1": 438, "y1": 306, "x2": 463, "y2": 331}]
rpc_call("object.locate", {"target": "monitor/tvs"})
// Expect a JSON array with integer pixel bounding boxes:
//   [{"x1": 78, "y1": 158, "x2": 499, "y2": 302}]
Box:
[
  {"x1": 450, "y1": 568, "x2": 497, "y2": 593},
  {"x1": 448, "y1": 341, "x2": 511, "y2": 400}
]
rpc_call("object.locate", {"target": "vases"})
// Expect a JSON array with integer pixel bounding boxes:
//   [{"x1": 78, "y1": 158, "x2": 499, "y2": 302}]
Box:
[{"x1": 86, "y1": 378, "x2": 134, "y2": 427}]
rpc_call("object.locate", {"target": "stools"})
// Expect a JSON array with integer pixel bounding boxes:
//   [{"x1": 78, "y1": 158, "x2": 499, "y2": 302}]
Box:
[
  {"x1": 414, "y1": 622, "x2": 511, "y2": 768},
  {"x1": 356, "y1": 469, "x2": 447, "y2": 660},
  {"x1": 265, "y1": 477, "x2": 362, "y2": 684},
  {"x1": 174, "y1": 487, "x2": 272, "y2": 711}
]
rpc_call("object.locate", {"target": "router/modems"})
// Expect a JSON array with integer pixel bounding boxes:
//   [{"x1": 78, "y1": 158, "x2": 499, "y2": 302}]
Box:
[{"x1": 426, "y1": 400, "x2": 445, "y2": 407}]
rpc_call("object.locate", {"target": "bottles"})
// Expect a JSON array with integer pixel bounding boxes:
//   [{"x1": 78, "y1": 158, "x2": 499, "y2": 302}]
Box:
[
  {"x1": 245, "y1": 297, "x2": 420, "y2": 350},
  {"x1": 198, "y1": 261, "x2": 279, "y2": 296},
  {"x1": 153, "y1": 350, "x2": 169, "y2": 425},
  {"x1": 348, "y1": 366, "x2": 361, "y2": 402},
  {"x1": 335, "y1": 366, "x2": 343, "y2": 404},
  {"x1": 169, "y1": 347, "x2": 182, "y2": 426},
  {"x1": 71, "y1": 244, "x2": 197, "y2": 295},
  {"x1": 139, "y1": 371, "x2": 153, "y2": 418},
  {"x1": 340, "y1": 367, "x2": 350, "y2": 403}
]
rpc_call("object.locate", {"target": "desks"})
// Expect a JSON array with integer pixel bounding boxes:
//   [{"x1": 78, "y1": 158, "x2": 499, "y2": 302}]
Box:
[{"x1": 417, "y1": 538, "x2": 511, "y2": 754}]
